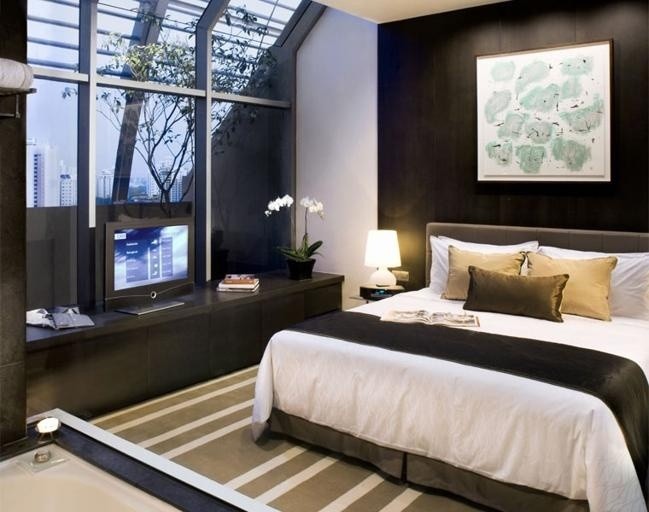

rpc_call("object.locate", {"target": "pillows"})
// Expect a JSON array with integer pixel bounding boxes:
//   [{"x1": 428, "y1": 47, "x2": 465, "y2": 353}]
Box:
[
  {"x1": 524, "y1": 247, "x2": 619, "y2": 323},
  {"x1": 540, "y1": 245, "x2": 649, "y2": 324},
  {"x1": 437, "y1": 244, "x2": 528, "y2": 302},
  {"x1": 426, "y1": 236, "x2": 538, "y2": 298},
  {"x1": 464, "y1": 264, "x2": 569, "y2": 324}
]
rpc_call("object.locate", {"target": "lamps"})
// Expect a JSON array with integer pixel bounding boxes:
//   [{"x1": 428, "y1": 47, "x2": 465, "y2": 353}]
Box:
[{"x1": 362, "y1": 224, "x2": 400, "y2": 288}]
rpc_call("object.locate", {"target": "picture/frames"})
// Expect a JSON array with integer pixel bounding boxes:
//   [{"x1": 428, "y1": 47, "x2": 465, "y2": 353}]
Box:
[{"x1": 472, "y1": 37, "x2": 616, "y2": 186}]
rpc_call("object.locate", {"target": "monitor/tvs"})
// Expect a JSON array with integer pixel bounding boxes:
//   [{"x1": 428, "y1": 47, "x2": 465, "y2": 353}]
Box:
[{"x1": 104, "y1": 217, "x2": 196, "y2": 316}]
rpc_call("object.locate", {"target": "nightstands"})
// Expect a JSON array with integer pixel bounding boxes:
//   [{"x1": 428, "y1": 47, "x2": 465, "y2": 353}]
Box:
[{"x1": 359, "y1": 286, "x2": 404, "y2": 303}]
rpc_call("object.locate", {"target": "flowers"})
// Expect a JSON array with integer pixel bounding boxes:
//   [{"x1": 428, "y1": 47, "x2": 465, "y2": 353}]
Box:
[{"x1": 257, "y1": 190, "x2": 329, "y2": 261}]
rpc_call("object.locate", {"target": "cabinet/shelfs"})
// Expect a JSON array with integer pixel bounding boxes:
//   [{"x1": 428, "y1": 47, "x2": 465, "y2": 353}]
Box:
[{"x1": 25, "y1": 265, "x2": 345, "y2": 424}]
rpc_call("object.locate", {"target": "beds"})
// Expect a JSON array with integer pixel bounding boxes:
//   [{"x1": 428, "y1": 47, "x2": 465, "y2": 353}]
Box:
[{"x1": 253, "y1": 222, "x2": 649, "y2": 512}]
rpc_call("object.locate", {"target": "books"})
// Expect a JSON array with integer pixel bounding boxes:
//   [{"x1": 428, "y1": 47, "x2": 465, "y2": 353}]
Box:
[
  {"x1": 380, "y1": 310, "x2": 480, "y2": 326},
  {"x1": 216, "y1": 274, "x2": 260, "y2": 293}
]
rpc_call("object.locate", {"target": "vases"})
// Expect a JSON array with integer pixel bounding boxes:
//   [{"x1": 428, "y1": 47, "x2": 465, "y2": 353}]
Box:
[{"x1": 287, "y1": 257, "x2": 319, "y2": 279}]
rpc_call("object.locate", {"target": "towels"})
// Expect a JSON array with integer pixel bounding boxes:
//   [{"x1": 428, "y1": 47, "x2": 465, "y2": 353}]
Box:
[{"x1": 1, "y1": 58, "x2": 34, "y2": 90}]
